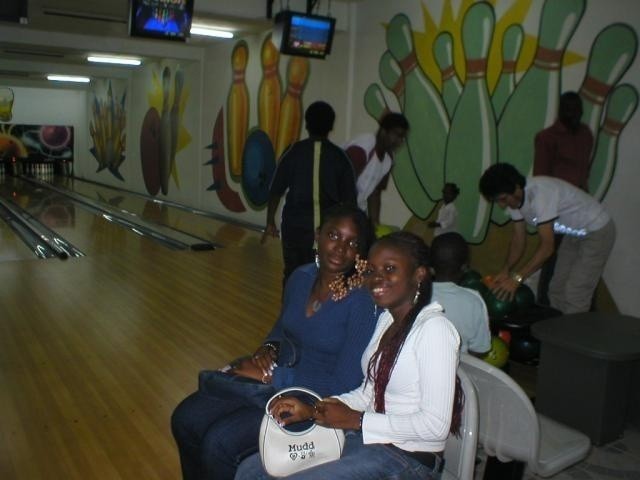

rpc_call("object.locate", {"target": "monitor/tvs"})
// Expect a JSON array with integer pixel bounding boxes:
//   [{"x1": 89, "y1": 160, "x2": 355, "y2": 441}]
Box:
[
  {"x1": 127, "y1": 0, "x2": 194, "y2": 44},
  {"x1": 280, "y1": 11, "x2": 339, "y2": 62}
]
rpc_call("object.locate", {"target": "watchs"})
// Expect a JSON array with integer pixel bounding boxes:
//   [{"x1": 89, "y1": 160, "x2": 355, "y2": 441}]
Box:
[{"x1": 512, "y1": 271, "x2": 526, "y2": 285}]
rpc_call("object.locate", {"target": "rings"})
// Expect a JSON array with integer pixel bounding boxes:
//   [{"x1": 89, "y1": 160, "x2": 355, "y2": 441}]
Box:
[{"x1": 253, "y1": 352, "x2": 261, "y2": 360}]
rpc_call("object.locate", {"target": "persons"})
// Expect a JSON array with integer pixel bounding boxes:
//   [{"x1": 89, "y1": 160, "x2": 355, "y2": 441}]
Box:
[
  {"x1": 426, "y1": 183, "x2": 461, "y2": 242},
  {"x1": 260, "y1": 99, "x2": 356, "y2": 303},
  {"x1": 170, "y1": 203, "x2": 384, "y2": 479},
  {"x1": 477, "y1": 162, "x2": 617, "y2": 316},
  {"x1": 415, "y1": 232, "x2": 536, "y2": 478},
  {"x1": 235, "y1": 231, "x2": 461, "y2": 479},
  {"x1": 340, "y1": 112, "x2": 410, "y2": 226},
  {"x1": 531, "y1": 88, "x2": 598, "y2": 314}
]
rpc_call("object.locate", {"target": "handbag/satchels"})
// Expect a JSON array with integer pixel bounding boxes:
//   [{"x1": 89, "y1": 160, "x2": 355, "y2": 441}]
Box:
[
  {"x1": 257, "y1": 386, "x2": 346, "y2": 479},
  {"x1": 198, "y1": 362, "x2": 296, "y2": 412}
]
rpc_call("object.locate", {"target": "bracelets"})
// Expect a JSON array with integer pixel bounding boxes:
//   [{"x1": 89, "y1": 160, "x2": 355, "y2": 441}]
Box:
[
  {"x1": 359, "y1": 412, "x2": 366, "y2": 432},
  {"x1": 264, "y1": 342, "x2": 278, "y2": 353}
]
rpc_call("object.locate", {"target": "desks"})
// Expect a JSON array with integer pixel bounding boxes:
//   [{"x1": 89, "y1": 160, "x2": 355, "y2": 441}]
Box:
[{"x1": 532, "y1": 313, "x2": 640, "y2": 446}]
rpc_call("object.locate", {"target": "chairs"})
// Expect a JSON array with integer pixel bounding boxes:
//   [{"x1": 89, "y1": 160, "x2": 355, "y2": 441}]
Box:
[
  {"x1": 442, "y1": 369, "x2": 480, "y2": 480},
  {"x1": 458, "y1": 353, "x2": 591, "y2": 480}
]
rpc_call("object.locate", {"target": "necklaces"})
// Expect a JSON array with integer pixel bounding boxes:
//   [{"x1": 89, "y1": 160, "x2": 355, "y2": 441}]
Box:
[{"x1": 311, "y1": 269, "x2": 338, "y2": 312}]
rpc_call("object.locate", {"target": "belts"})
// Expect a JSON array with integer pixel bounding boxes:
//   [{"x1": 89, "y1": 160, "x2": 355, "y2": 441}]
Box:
[{"x1": 410, "y1": 449, "x2": 447, "y2": 474}]
